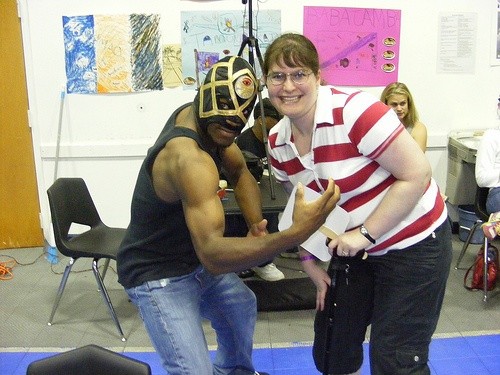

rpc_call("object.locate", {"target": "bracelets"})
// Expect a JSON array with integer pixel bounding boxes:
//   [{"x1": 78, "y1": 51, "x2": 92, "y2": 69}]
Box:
[{"x1": 299, "y1": 256, "x2": 316, "y2": 261}]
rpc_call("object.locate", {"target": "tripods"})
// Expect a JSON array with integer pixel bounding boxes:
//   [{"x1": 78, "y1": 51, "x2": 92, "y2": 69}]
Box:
[{"x1": 236, "y1": 0, "x2": 282, "y2": 200}]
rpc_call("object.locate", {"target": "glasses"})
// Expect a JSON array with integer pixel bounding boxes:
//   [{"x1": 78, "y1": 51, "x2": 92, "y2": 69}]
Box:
[{"x1": 264, "y1": 69, "x2": 316, "y2": 86}]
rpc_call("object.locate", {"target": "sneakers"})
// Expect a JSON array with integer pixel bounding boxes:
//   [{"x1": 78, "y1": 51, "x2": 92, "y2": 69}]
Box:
[
  {"x1": 251, "y1": 262, "x2": 286, "y2": 282},
  {"x1": 280, "y1": 250, "x2": 301, "y2": 259}
]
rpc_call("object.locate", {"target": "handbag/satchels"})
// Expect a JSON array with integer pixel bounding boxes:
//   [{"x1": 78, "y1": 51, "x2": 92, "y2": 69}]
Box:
[{"x1": 462, "y1": 242, "x2": 500, "y2": 290}]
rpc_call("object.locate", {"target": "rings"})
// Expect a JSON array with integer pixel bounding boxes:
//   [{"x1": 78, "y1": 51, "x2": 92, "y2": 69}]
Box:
[{"x1": 343, "y1": 251, "x2": 349, "y2": 256}]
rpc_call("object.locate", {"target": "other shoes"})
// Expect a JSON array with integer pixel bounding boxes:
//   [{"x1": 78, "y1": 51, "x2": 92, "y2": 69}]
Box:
[{"x1": 254, "y1": 371, "x2": 271, "y2": 375}]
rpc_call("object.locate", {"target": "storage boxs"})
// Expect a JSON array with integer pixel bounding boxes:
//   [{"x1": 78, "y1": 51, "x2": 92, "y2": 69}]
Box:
[{"x1": 457, "y1": 207, "x2": 484, "y2": 244}]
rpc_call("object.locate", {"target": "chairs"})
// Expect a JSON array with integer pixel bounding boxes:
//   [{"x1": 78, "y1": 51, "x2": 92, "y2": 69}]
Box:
[
  {"x1": 26, "y1": 344, "x2": 152, "y2": 375},
  {"x1": 454, "y1": 185, "x2": 500, "y2": 303},
  {"x1": 47, "y1": 177, "x2": 127, "y2": 342}
]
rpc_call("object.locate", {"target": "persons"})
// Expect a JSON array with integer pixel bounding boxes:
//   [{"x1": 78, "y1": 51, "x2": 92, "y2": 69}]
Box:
[
  {"x1": 380, "y1": 82, "x2": 427, "y2": 153},
  {"x1": 263, "y1": 34, "x2": 452, "y2": 375},
  {"x1": 234, "y1": 98, "x2": 284, "y2": 177},
  {"x1": 475, "y1": 97, "x2": 500, "y2": 215},
  {"x1": 116, "y1": 55, "x2": 341, "y2": 375}
]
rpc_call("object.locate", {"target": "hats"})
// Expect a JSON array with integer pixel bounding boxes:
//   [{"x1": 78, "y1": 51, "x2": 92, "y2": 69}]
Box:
[{"x1": 253, "y1": 97, "x2": 284, "y2": 120}]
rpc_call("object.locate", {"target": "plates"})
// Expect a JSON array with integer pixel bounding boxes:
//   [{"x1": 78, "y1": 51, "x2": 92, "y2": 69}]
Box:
[
  {"x1": 381, "y1": 62, "x2": 395, "y2": 73},
  {"x1": 381, "y1": 49, "x2": 396, "y2": 60},
  {"x1": 382, "y1": 37, "x2": 396, "y2": 47}
]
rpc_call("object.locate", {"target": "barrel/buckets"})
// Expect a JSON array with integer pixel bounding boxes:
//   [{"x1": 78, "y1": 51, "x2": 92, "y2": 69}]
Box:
[{"x1": 458, "y1": 204, "x2": 484, "y2": 244}]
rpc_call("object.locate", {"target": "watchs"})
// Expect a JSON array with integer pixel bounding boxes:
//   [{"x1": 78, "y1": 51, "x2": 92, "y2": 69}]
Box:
[{"x1": 360, "y1": 224, "x2": 375, "y2": 244}]
